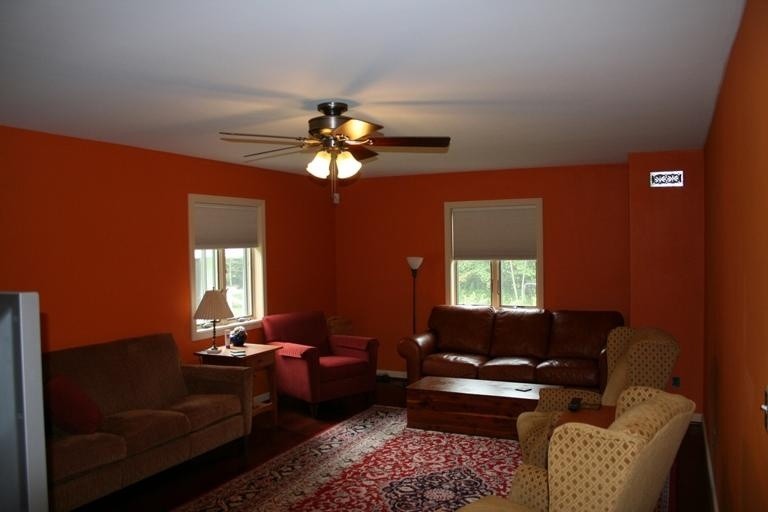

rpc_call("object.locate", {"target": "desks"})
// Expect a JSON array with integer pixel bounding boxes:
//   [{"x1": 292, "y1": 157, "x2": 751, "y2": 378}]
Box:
[
  {"x1": 554, "y1": 404, "x2": 615, "y2": 430},
  {"x1": 194, "y1": 342, "x2": 284, "y2": 424}
]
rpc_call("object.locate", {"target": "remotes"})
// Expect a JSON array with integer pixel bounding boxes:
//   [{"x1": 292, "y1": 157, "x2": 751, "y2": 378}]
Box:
[{"x1": 569, "y1": 398, "x2": 582, "y2": 412}]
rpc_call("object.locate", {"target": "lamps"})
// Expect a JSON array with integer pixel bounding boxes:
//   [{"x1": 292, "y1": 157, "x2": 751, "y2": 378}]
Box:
[
  {"x1": 404, "y1": 255, "x2": 423, "y2": 335},
  {"x1": 304, "y1": 138, "x2": 362, "y2": 205},
  {"x1": 192, "y1": 287, "x2": 234, "y2": 354}
]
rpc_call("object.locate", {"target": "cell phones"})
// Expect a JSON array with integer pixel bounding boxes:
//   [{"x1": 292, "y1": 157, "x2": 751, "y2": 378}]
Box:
[
  {"x1": 231, "y1": 353, "x2": 245, "y2": 356},
  {"x1": 230, "y1": 350, "x2": 245, "y2": 353}
]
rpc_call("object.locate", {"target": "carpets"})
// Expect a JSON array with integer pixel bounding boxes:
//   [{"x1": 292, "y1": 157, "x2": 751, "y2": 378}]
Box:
[{"x1": 173, "y1": 404, "x2": 521, "y2": 512}]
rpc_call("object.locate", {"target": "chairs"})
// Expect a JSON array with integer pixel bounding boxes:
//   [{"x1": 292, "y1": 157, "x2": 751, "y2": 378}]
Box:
[
  {"x1": 453, "y1": 385, "x2": 696, "y2": 512},
  {"x1": 515, "y1": 324, "x2": 680, "y2": 467},
  {"x1": 260, "y1": 309, "x2": 378, "y2": 417}
]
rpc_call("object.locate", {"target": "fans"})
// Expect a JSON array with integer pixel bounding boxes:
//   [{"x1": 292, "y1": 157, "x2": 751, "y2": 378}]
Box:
[{"x1": 218, "y1": 99, "x2": 451, "y2": 159}]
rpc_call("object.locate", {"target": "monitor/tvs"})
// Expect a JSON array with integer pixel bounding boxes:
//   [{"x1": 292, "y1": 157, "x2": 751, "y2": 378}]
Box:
[{"x1": 0, "y1": 291, "x2": 50, "y2": 512}]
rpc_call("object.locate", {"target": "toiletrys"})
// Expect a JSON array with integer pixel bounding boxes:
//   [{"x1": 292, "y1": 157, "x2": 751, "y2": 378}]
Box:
[{"x1": 224, "y1": 329, "x2": 230, "y2": 348}]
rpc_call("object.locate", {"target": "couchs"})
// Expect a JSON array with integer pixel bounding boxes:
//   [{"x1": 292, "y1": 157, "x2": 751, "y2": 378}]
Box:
[
  {"x1": 395, "y1": 303, "x2": 624, "y2": 389},
  {"x1": 42, "y1": 330, "x2": 255, "y2": 511}
]
